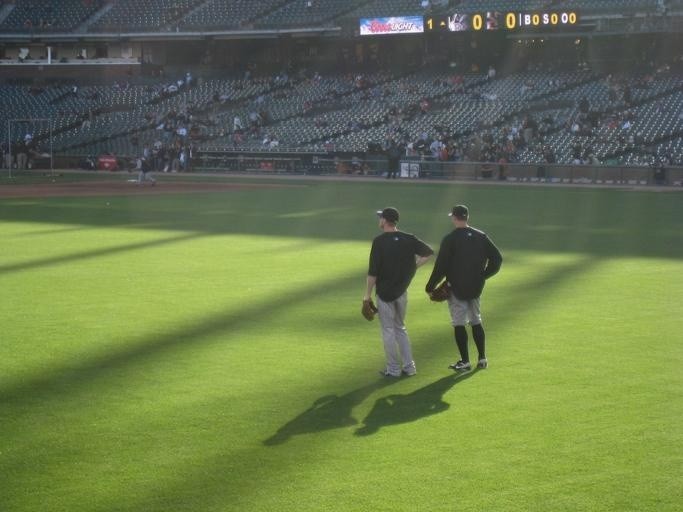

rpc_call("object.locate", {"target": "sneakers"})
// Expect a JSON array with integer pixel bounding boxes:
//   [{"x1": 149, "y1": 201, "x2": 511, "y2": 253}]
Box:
[
  {"x1": 381, "y1": 360, "x2": 417, "y2": 377},
  {"x1": 447, "y1": 359, "x2": 471, "y2": 370},
  {"x1": 476, "y1": 359, "x2": 488, "y2": 369}
]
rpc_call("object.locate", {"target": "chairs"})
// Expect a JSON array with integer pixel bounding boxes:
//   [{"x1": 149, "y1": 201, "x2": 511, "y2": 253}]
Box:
[{"x1": 0, "y1": 0, "x2": 683, "y2": 168}]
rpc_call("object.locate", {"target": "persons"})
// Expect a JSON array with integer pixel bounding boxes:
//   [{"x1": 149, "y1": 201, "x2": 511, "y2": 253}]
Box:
[
  {"x1": 20, "y1": 16, "x2": 58, "y2": 33},
  {"x1": 423, "y1": 204, "x2": 504, "y2": 372},
  {"x1": 361, "y1": 206, "x2": 434, "y2": 378},
  {"x1": 0, "y1": 61, "x2": 682, "y2": 188}
]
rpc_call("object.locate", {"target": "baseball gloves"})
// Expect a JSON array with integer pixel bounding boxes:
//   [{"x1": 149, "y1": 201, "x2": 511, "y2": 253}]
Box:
[
  {"x1": 362, "y1": 298, "x2": 378, "y2": 321},
  {"x1": 430, "y1": 281, "x2": 450, "y2": 301}
]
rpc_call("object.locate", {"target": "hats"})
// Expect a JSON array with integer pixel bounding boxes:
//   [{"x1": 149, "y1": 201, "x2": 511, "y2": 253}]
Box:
[
  {"x1": 448, "y1": 205, "x2": 469, "y2": 217},
  {"x1": 377, "y1": 207, "x2": 400, "y2": 224}
]
